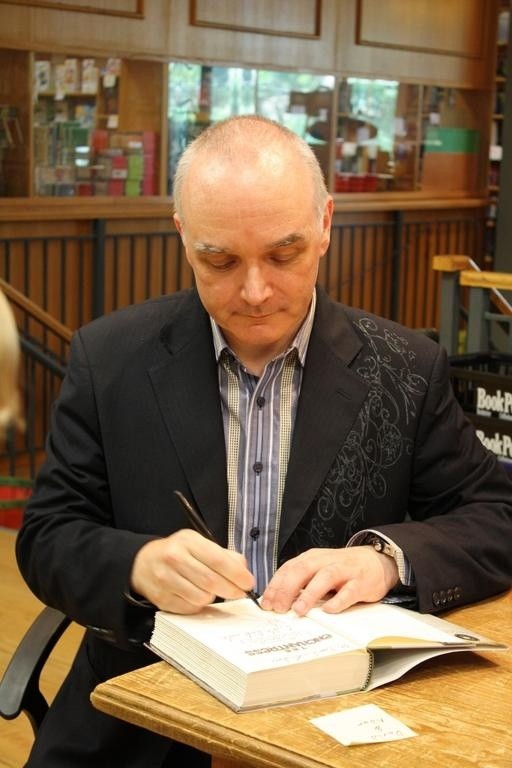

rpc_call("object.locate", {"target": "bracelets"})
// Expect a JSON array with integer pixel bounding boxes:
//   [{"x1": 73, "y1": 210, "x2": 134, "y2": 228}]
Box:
[{"x1": 370, "y1": 538, "x2": 395, "y2": 558}]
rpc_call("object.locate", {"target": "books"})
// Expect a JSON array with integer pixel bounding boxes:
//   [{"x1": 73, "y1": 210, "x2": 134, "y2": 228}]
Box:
[{"x1": 139, "y1": 582, "x2": 509, "y2": 713}]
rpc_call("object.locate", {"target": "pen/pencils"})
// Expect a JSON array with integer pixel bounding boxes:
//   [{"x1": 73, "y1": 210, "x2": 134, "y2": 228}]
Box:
[{"x1": 174, "y1": 490, "x2": 263, "y2": 610}]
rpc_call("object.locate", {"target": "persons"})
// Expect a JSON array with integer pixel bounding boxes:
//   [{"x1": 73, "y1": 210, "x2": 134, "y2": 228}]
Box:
[{"x1": 12, "y1": 114, "x2": 510, "y2": 768}]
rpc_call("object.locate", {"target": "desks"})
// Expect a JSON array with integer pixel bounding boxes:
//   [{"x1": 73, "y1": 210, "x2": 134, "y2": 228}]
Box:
[{"x1": 85, "y1": 586, "x2": 509, "y2": 766}]
